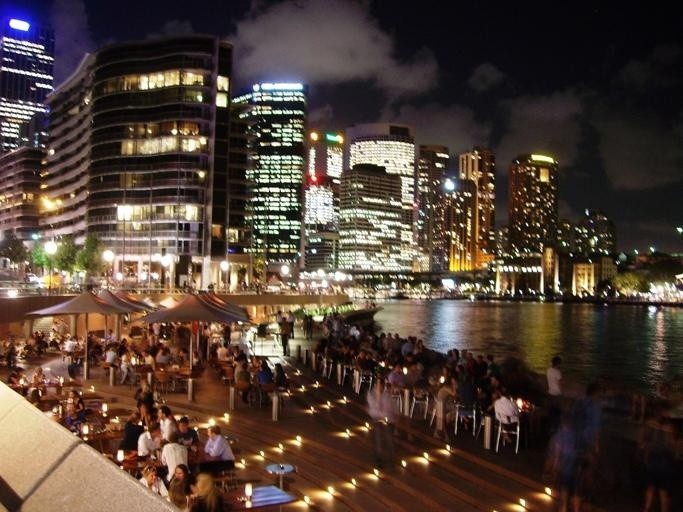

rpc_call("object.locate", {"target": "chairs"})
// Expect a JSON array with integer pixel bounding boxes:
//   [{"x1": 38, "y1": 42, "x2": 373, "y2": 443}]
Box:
[{"x1": 313, "y1": 337, "x2": 521, "y2": 455}]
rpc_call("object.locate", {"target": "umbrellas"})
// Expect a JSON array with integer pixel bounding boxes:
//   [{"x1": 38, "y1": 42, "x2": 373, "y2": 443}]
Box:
[
  {"x1": 92, "y1": 287, "x2": 145, "y2": 348},
  {"x1": 24, "y1": 288, "x2": 137, "y2": 381},
  {"x1": 114, "y1": 289, "x2": 252, "y2": 372}
]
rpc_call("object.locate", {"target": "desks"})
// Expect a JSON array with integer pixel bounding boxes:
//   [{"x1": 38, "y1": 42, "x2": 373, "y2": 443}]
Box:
[{"x1": 0, "y1": 327, "x2": 291, "y2": 508}]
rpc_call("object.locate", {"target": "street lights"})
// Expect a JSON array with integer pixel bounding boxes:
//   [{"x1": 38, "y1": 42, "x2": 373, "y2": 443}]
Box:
[
  {"x1": 116, "y1": 204, "x2": 129, "y2": 287},
  {"x1": 101, "y1": 249, "x2": 114, "y2": 289},
  {"x1": 162, "y1": 253, "x2": 175, "y2": 287},
  {"x1": 280, "y1": 266, "x2": 290, "y2": 291},
  {"x1": 216, "y1": 260, "x2": 229, "y2": 290},
  {"x1": 44, "y1": 240, "x2": 58, "y2": 294}
]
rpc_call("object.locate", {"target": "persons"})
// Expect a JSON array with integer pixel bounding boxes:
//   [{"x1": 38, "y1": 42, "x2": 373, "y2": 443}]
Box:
[
  {"x1": 276, "y1": 310, "x2": 283, "y2": 325},
  {"x1": 277, "y1": 316, "x2": 291, "y2": 357},
  {"x1": 542, "y1": 354, "x2": 683, "y2": 512},
  {"x1": 304, "y1": 309, "x2": 526, "y2": 443},
  {"x1": 3, "y1": 322, "x2": 290, "y2": 512},
  {"x1": 288, "y1": 311, "x2": 296, "y2": 339}
]
